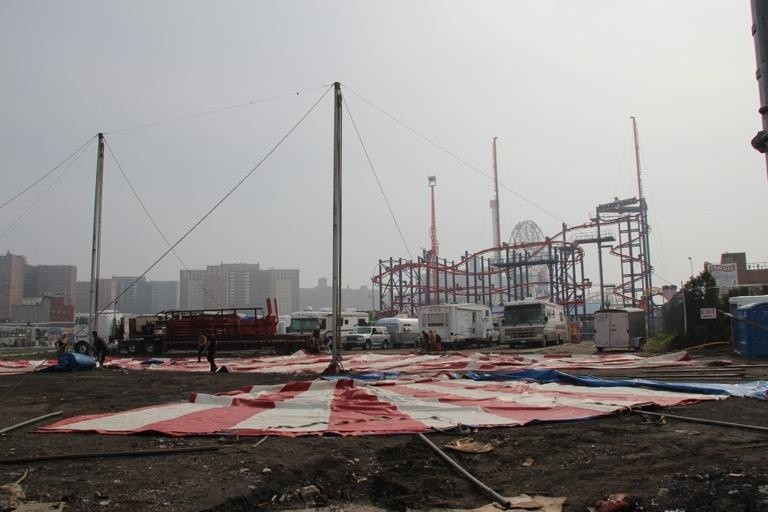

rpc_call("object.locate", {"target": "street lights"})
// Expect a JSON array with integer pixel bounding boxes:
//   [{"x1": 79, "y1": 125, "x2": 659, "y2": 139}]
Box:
[
  {"x1": 688, "y1": 255, "x2": 694, "y2": 280},
  {"x1": 427, "y1": 173, "x2": 440, "y2": 304}
]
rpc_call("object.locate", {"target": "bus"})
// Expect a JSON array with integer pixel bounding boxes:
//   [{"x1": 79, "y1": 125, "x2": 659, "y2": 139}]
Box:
[
  {"x1": 287, "y1": 306, "x2": 370, "y2": 347},
  {"x1": 503, "y1": 300, "x2": 569, "y2": 348},
  {"x1": 374, "y1": 317, "x2": 418, "y2": 347}
]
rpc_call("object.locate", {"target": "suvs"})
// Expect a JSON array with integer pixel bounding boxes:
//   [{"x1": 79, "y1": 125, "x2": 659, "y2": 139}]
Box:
[{"x1": 341, "y1": 326, "x2": 391, "y2": 350}]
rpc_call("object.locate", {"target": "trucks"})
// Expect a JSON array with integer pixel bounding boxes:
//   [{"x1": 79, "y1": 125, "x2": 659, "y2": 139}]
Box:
[
  {"x1": 71, "y1": 298, "x2": 279, "y2": 355},
  {"x1": 416, "y1": 303, "x2": 500, "y2": 350}
]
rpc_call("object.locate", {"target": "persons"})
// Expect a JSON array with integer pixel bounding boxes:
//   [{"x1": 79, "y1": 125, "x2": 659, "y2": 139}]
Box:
[
  {"x1": 420, "y1": 330, "x2": 430, "y2": 352},
  {"x1": 91, "y1": 329, "x2": 109, "y2": 367},
  {"x1": 55, "y1": 333, "x2": 69, "y2": 351},
  {"x1": 434, "y1": 330, "x2": 443, "y2": 350},
  {"x1": 196, "y1": 331, "x2": 207, "y2": 362},
  {"x1": 204, "y1": 328, "x2": 218, "y2": 373},
  {"x1": 427, "y1": 330, "x2": 441, "y2": 354}
]
